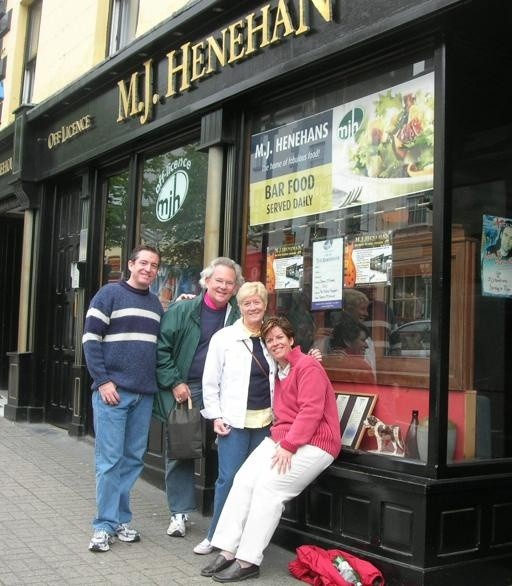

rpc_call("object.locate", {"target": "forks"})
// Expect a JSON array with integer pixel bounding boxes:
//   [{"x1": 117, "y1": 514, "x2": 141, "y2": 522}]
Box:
[{"x1": 333, "y1": 185, "x2": 364, "y2": 208}]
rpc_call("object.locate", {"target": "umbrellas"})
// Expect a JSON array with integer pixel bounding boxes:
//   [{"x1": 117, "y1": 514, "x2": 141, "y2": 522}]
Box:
[
  {"x1": 201, "y1": 554, "x2": 235, "y2": 576},
  {"x1": 212, "y1": 561, "x2": 260, "y2": 582}
]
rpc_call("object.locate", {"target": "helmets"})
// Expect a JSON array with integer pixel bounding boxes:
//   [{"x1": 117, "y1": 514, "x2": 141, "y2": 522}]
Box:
[{"x1": 343, "y1": 160, "x2": 434, "y2": 185}]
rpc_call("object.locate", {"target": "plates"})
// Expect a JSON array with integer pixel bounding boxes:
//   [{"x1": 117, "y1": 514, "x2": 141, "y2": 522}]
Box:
[{"x1": 312, "y1": 222, "x2": 512, "y2": 393}]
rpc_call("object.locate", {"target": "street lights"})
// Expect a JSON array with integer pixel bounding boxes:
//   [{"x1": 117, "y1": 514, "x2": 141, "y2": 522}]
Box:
[{"x1": 166, "y1": 399, "x2": 207, "y2": 459}]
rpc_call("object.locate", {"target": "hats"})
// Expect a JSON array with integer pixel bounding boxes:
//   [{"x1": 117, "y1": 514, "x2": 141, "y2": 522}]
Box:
[{"x1": 385, "y1": 317, "x2": 433, "y2": 363}]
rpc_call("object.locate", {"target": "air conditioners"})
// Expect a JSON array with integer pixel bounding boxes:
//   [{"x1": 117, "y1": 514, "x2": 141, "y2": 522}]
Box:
[{"x1": 362, "y1": 415, "x2": 404, "y2": 455}]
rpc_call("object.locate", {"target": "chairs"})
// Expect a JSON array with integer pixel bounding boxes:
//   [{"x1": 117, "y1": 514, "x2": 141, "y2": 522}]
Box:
[
  {"x1": 89, "y1": 528, "x2": 113, "y2": 551},
  {"x1": 193, "y1": 539, "x2": 214, "y2": 555},
  {"x1": 115, "y1": 523, "x2": 140, "y2": 542},
  {"x1": 167, "y1": 513, "x2": 186, "y2": 537}
]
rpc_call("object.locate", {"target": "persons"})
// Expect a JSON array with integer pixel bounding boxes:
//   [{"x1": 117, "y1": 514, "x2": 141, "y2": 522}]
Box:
[
  {"x1": 328, "y1": 290, "x2": 371, "y2": 337},
  {"x1": 199, "y1": 313, "x2": 342, "y2": 583},
  {"x1": 151, "y1": 256, "x2": 245, "y2": 538},
  {"x1": 323, "y1": 319, "x2": 374, "y2": 378},
  {"x1": 192, "y1": 281, "x2": 324, "y2": 558},
  {"x1": 487, "y1": 220, "x2": 511, "y2": 262},
  {"x1": 79, "y1": 244, "x2": 199, "y2": 555}
]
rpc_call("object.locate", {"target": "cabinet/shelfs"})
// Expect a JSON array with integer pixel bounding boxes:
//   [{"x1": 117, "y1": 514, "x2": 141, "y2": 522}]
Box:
[{"x1": 331, "y1": 555, "x2": 364, "y2": 586}]
